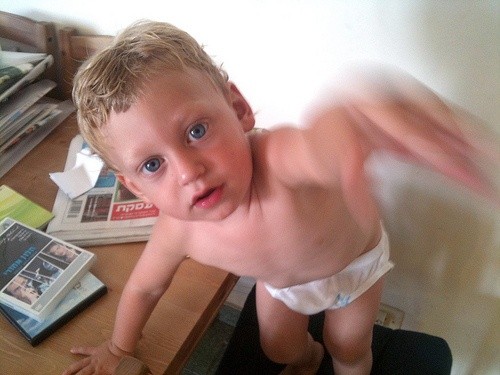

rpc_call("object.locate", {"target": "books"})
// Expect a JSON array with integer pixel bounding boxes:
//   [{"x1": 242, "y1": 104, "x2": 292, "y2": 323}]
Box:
[
  {"x1": 0, "y1": 269, "x2": 109, "y2": 348},
  {"x1": 1, "y1": 184, "x2": 55, "y2": 233},
  {"x1": 0, "y1": 218, "x2": 97, "y2": 323}
]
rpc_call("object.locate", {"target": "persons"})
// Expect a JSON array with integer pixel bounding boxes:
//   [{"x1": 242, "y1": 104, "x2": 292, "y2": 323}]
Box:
[
  {"x1": 46, "y1": 243, "x2": 81, "y2": 263},
  {"x1": 6, "y1": 275, "x2": 42, "y2": 306},
  {"x1": 58, "y1": 17, "x2": 500, "y2": 375}
]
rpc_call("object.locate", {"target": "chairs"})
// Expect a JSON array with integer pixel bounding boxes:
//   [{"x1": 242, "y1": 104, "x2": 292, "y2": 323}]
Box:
[
  {"x1": 0, "y1": 10, "x2": 60, "y2": 85},
  {"x1": 59, "y1": 24, "x2": 117, "y2": 103},
  {"x1": 213, "y1": 285, "x2": 455, "y2": 375}
]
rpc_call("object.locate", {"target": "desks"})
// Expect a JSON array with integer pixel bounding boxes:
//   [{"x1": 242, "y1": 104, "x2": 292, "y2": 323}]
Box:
[{"x1": 0, "y1": 94, "x2": 244, "y2": 374}]
